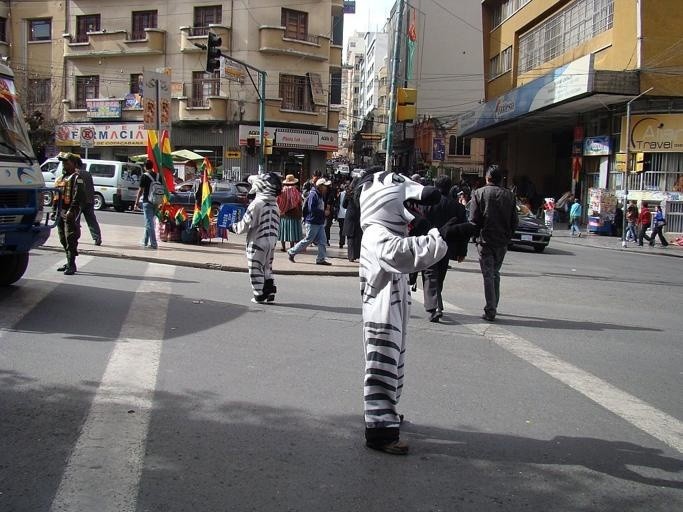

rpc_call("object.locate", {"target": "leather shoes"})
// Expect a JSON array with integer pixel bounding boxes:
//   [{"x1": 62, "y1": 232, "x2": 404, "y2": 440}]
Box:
[
  {"x1": 56, "y1": 263, "x2": 75, "y2": 272},
  {"x1": 429, "y1": 308, "x2": 442, "y2": 322},
  {"x1": 63, "y1": 268, "x2": 74, "y2": 275}
]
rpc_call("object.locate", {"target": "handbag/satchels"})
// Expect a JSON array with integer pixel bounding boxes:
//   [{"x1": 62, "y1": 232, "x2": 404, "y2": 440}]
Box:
[{"x1": 655, "y1": 219, "x2": 666, "y2": 228}]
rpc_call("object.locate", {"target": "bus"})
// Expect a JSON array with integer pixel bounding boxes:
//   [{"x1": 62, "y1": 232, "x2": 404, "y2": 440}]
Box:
[{"x1": 0, "y1": 64, "x2": 50, "y2": 285}]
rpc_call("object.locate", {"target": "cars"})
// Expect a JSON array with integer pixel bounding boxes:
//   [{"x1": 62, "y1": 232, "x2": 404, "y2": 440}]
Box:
[
  {"x1": 462, "y1": 199, "x2": 552, "y2": 252},
  {"x1": 325, "y1": 155, "x2": 366, "y2": 181}
]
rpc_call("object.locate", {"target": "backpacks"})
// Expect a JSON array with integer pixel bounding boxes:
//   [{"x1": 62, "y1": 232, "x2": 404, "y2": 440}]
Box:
[{"x1": 143, "y1": 172, "x2": 164, "y2": 205}]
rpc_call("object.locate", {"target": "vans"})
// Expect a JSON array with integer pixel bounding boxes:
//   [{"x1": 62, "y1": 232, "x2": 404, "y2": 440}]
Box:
[
  {"x1": 137, "y1": 176, "x2": 254, "y2": 218},
  {"x1": 39, "y1": 158, "x2": 142, "y2": 212}
]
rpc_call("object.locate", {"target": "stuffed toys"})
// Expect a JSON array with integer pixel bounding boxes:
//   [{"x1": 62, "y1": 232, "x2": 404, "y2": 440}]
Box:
[
  {"x1": 229, "y1": 169, "x2": 282, "y2": 303},
  {"x1": 543, "y1": 197, "x2": 556, "y2": 232},
  {"x1": 352, "y1": 169, "x2": 478, "y2": 458}
]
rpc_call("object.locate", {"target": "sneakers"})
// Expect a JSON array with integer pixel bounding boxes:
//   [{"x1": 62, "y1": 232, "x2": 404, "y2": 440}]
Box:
[
  {"x1": 287, "y1": 252, "x2": 295, "y2": 263},
  {"x1": 316, "y1": 261, "x2": 330, "y2": 265}
]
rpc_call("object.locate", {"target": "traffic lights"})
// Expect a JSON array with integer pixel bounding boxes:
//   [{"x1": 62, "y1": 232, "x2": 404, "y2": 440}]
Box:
[
  {"x1": 636, "y1": 152, "x2": 652, "y2": 174},
  {"x1": 245, "y1": 137, "x2": 256, "y2": 155},
  {"x1": 204, "y1": 31, "x2": 222, "y2": 74},
  {"x1": 262, "y1": 137, "x2": 275, "y2": 156},
  {"x1": 395, "y1": 85, "x2": 416, "y2": 124}
]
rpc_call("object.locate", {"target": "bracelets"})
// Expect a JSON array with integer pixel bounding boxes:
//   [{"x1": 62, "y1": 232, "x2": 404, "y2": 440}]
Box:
[{"x1": 135, "y1": 202, "x2": 139, "y2": 206}]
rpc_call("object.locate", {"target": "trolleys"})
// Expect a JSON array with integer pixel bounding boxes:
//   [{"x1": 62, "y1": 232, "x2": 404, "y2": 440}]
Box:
[{"x1": 585, "y1": 187, "x2": 617, "y2": 237}]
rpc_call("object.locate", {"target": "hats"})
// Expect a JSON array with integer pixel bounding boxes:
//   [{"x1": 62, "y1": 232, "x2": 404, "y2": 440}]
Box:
[
  {"x1": 57, "y1": 153, "x2": 76, "y2": 162},
  {"x1": 282, "y1": 174, "x2": 298, "y2": 184},
  {"x1": 315, "y1": 178, "x2": 331, "y2": 185}
]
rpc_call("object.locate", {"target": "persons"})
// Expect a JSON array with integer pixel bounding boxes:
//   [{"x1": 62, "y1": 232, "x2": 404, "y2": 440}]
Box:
[
  {"x1": 73, "y1": 154, "x2": 102, "y2": 246},
  {"x1": 625, "y1": 200, "x2": 637, "y2": 244},
  {"x1": 277, "y1": 164, "x2": 518, "y2": 322},
  {"x1": 133, "y1": 160, "x2": 163, "y2": 250},
  {"x1": 565, "y1": 194, "x2": 574, "y2": 230},
  {"x1": 636, "y1": 200, "x2": 655, "y2": 246},
  {"x1": 649, "y1": 206, "x2": 669, "y2": 247},
  {"x1": 569, "y1": 199, "x2": 582, "y2": 237},
  {"x1": 48, "y1": 153, "x2": 85, "y2": 275}
]
